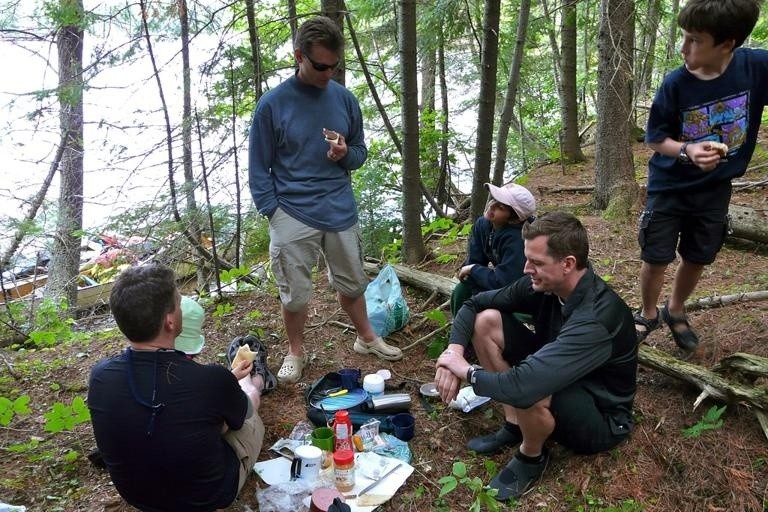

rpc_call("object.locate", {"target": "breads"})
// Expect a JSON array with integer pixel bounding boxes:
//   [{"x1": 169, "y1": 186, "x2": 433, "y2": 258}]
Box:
[
  {"x1": 323, "y1": 128, "x2": 340, "y2": 145},
  {"x1": 231, "y1": 343, "x2": 258, "y2": 370},
  {"x1": 705, "y1": 140, "x2": 729, "y2": 159}
]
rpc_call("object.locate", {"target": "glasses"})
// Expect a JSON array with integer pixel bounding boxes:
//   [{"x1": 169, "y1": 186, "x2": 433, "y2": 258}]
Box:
[{"x1": 304, "y1": 53, "x2": 340, "y2": 71}]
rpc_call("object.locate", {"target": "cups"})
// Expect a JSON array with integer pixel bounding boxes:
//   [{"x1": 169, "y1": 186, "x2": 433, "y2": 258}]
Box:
[
  {"x1": 385, "y1": 413, "x2": 415, "y2": 442},
  {"x1": 288, "y1": 445, "x2": 322, "y2": 483},
  {"x1": 336, "y1": 369, "x2": 361, "y2": 391},
  {"x1": 362, "y1": 374, "x2": 384, "y2": 409},
  {"x1": 303, "y1": 427, "x2": 333, "y2": 453},
  {"x1": 306, "y1": 489, "x2": 345, "y2": 512}
]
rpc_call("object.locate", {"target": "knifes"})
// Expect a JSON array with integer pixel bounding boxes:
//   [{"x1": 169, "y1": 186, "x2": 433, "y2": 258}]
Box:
[{"x1": 357, "y1": 463, "x2": 402, "y2": 498}]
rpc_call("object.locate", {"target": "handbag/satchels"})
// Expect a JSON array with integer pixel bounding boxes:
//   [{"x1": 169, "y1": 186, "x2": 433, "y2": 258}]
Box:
[{"x1": 303, "y1": 369, "x2": 368, "y2": 412}]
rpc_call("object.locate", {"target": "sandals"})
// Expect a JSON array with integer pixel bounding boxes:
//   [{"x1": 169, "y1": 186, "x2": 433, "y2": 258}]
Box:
[
  {"x1": 354, "y1": 336, "x2": 402, "y2": 361},
  {"x1": 278, "y1": 345, "x2": 307, "y2": 384}
]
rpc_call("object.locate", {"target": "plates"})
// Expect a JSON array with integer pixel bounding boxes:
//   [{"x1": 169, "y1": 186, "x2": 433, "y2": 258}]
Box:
[
  {"x1": 418, "y1": 382, "x2": 440, "y2": 397},
  {"x1": 309, "y1": 389, "x2": 367, "y2": 412}
]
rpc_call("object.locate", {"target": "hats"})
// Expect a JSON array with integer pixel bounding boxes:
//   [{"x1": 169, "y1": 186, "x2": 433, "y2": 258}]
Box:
[
  {"x1": 484, "y1": 182, "x2": 535, "y2": 220},
  {"x1": 175, "y1": 296, "x2": 205, "y2": 355}
]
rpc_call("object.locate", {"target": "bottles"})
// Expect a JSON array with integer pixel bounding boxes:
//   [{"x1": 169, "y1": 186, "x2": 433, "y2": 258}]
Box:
[
  {"x1": 365, "y1": 391, "x2": 412, "y2": 411},
  {"x1": 332, "y1": 410, "x2": 354, "y2": 452},
  {"x1": 327, "y1": 496, "x2": 352, "y2": 512}
]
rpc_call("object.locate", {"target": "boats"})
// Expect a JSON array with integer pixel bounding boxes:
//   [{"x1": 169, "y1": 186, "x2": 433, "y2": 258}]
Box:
[
  {"x1": 0, "y1": 222, "x2": 235, "y2": 323},
  {"x1": 0, "y1": 223, "x2": 151, "y2": 304}
]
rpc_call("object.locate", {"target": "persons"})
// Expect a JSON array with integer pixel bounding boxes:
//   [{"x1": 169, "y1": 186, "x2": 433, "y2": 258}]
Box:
[
  {"x1": 248, "y1": 15, "x2": 404, "y2": 385},
  {"x1": 434, "y1": 212, "x2": 638, "y2": 504},
  {"x1": 87, "y1": 263, "x2": 280, "y2": 512},
  {"x1": 451, "y1": 182, "x2": 537, "y2": 370},
  {"x1": 633, "y1": 0, "x2": 768, "y2": 353}
]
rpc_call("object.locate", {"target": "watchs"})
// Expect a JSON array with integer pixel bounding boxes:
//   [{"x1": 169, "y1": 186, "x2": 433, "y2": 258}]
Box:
[
  {"x1": 466, "y1": 362, "x2": 483, "y2": 383},
  {"x1": 678, "y1": 141, "x2": 691, "y2": 162}
]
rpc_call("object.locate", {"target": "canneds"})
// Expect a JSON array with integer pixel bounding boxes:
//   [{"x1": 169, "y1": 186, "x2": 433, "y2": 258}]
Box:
[
  {"x1": 311, "y1": 488, "x2": 345, "y2": 512},
  {"x1": 334, "y1": 450, "x2": 355, "y2": 491}
]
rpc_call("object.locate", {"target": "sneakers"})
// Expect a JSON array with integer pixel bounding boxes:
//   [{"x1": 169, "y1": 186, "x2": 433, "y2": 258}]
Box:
[
  {"x1": 486, "y1": 458, "x2": 543, "y2": 501},
  {"x1": 662, "y1": 301, "x2": 698, "y2": 350},
  {"x1": 228, "y1": 336, "x2": 278, "y2": 397},
  {"x1": 468, "y1": 426, "x2": 517, "y2": 457},
  {"x1": 633, "y1": 305, "x2": 662, "y2": 344}
]
